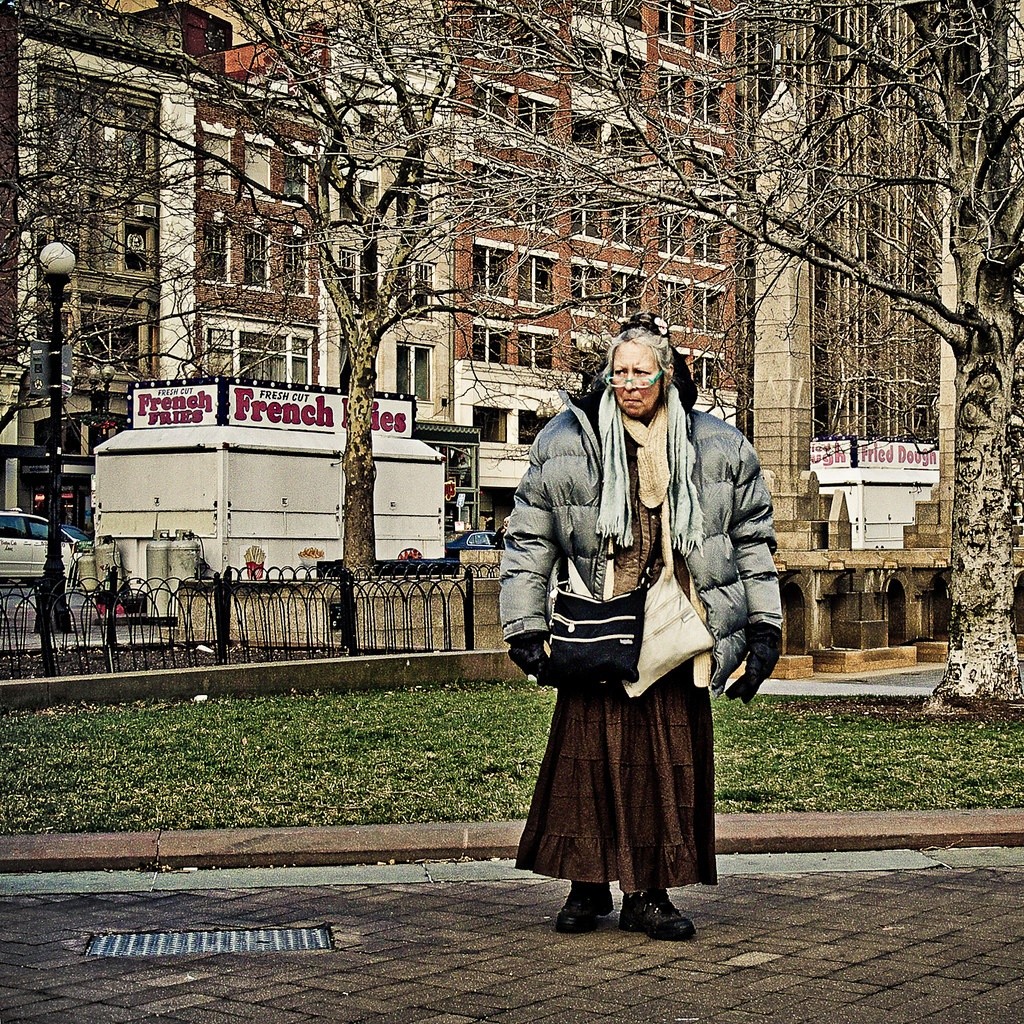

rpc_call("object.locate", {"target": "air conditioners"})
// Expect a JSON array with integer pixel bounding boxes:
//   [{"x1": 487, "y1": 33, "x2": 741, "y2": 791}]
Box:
[{"x1": 134, "y1": 203, "x2": 157, "y2": 218}]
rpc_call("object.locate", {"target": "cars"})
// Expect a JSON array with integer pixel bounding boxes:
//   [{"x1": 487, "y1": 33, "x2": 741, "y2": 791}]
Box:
[
  {"x1": 445, "y1": 529, "x2": 505, "y2": 558},
  {"x1": 0, "y1": 507, "x2": 93, "y2": 588}
]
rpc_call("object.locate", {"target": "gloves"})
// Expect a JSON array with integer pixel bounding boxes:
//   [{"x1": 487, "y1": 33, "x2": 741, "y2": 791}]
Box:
[
  {"x1": 726, "y1": 622, "x2": 782, "y2": 704},
  {"x1": 508, "y1": 636, "x2": 547, "y2": 678}
]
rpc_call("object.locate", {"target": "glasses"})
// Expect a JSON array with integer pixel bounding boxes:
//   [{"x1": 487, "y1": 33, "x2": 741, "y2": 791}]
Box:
[{"x1": 605, "y1": 370, "x2": 662, "y2": 389}]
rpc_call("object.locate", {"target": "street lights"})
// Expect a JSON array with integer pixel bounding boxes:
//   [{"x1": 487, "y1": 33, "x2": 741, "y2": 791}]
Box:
[{"x1": 33, "y1": 241, "x2": 78, "y2": 635}]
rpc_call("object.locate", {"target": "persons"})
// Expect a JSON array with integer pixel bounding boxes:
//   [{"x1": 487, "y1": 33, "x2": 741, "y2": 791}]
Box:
[{"x1": 498, "y1": 312, "x2": 785, "y2": 941}]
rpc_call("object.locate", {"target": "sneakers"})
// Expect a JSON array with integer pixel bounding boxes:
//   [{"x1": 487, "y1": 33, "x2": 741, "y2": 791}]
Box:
[
  {"x1": 619, "y1": 890, "x2": 696, "y2": 938},
  {"x1": 555, "y1": 881, "x2": 613, "y2": 933}
]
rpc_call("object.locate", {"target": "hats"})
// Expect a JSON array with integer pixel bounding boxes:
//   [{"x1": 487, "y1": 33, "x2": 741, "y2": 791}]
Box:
[{"x1": 620, "y1": 312, "x2": 670, "y2": 339}]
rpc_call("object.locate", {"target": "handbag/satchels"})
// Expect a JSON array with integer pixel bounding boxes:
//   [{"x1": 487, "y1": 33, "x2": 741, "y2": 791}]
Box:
[
  {"x1": 621, "y1": 567, "x2": 718, "y2": 697},
  {"x1": 537, "y1": 584, "x2": 647, "y2": 686}
]
